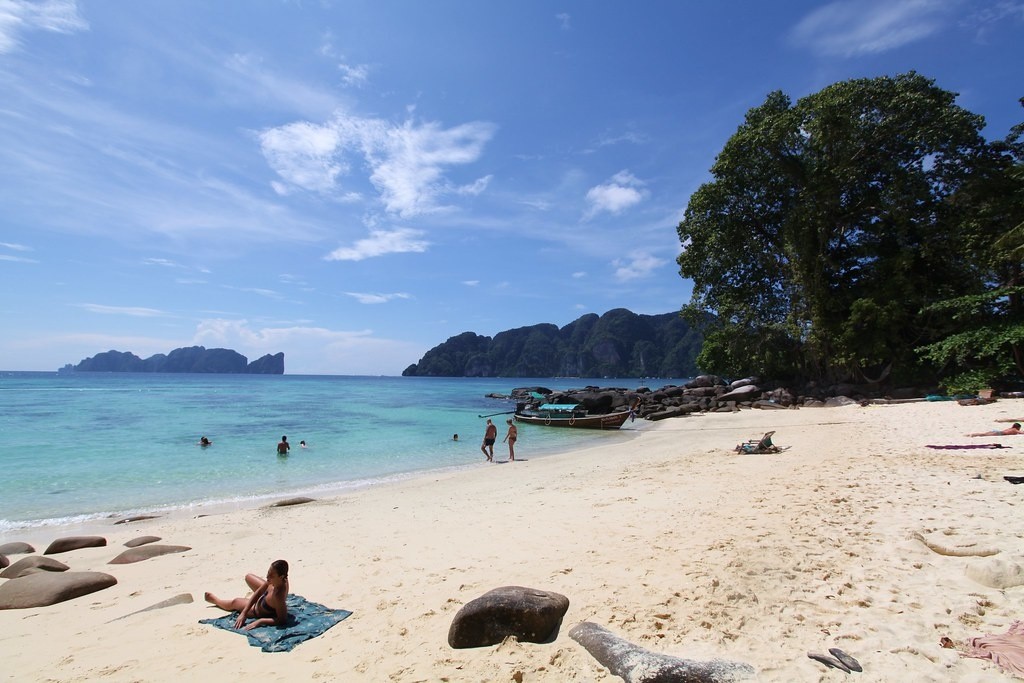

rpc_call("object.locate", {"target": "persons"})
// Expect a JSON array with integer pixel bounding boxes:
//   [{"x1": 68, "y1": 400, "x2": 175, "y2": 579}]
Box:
[
  {"x1": 503, "y1": 419, "x2": 517, "y2": 461},
  {"x1": 995, "y1": 417, "x2": 1024, "y2": 422},
  {"x1": 453, "y1": 434, "x2": 458, "y2": 441},
  {"x1": 204, "y1": 560, "x2": 289, "y2": 630},
  {"x1": 198, "y1": 437, "x2": 212, "y2": 444},
  {"x1": 277, "y1": 436, "x2": 290, "y2": 454},
  {"x1": 963, "y1": 423, "x2": 1024, "y2": 438},
  {"x1": 481, "y1": 419, "x2": 497, "y2": 462}
]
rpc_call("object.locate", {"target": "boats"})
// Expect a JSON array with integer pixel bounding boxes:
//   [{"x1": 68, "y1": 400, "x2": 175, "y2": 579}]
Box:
[
  {"x1": 515, "y1": 392, "x2": 640, "y2": 429},
  {"x1": 1000, "y1": 391, "x2": 1024, "y2": 398},
  {"x1": 958, "y1": 388, "x2": 1000, "y2": 406},
  {"x1": 926, "y1": 395, "x2": 976, "y2": 401}
]
